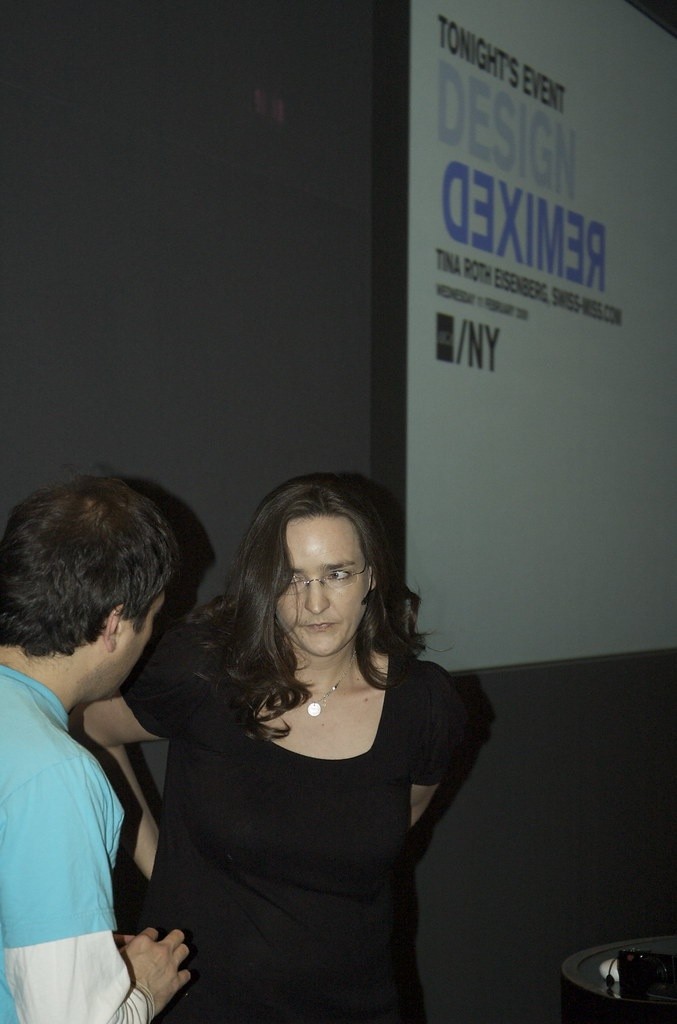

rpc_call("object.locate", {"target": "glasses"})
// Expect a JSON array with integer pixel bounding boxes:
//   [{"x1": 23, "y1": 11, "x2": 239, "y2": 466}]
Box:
[{"x1": 282, "y1": 562, "x2": 367, "y2": 595}]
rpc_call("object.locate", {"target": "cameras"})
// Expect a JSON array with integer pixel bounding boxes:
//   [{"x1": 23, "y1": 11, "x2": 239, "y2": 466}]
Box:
[{"x1": 618, "y1": 948, "x2": 677, "y2": 999}]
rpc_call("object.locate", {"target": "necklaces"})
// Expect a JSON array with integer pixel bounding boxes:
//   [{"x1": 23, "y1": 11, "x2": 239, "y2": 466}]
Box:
[{"x1": 302, "y1": 647, "x2": 357, "y2": 718}]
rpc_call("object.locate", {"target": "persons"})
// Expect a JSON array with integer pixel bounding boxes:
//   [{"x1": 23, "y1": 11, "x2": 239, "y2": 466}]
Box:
[
  {"x1": 68, "y1": 471, "x2": 470, "y2": 1024},
  {"x1": 0, "y1": 477, "x2": 191, "y2": 1024}
]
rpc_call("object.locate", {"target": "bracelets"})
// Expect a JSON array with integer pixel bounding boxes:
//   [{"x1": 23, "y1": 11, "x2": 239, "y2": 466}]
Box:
[{"x1": 131, "y1": 979, "x2": 155, "y2": 1018}]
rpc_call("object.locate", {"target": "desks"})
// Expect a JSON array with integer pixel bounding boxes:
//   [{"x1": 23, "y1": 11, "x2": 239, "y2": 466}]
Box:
[{"x1": 560, "y1": 934, "x2": 677, "y2": 1024}]
